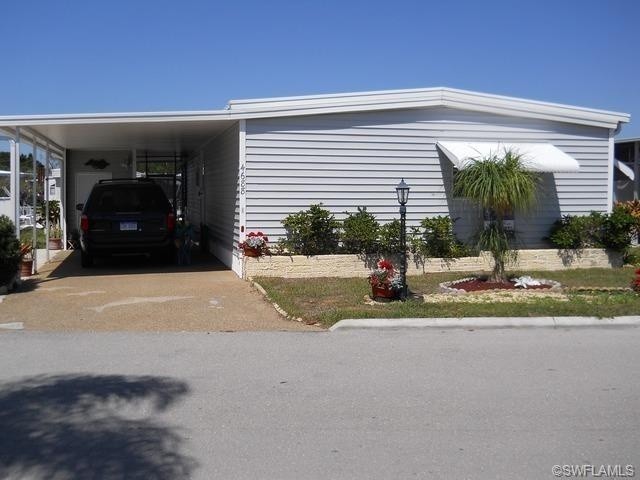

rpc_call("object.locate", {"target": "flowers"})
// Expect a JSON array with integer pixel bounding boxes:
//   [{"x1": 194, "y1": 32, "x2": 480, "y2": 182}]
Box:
[
  {"x1": 367, "y1": 260, "x2": 405, "y2": 291},
  {"x1": 238, "y1": 232, "x2": 268, "y2": 250}
]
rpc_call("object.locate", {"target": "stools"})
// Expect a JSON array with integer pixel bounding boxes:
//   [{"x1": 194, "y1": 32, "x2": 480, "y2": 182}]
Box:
[{"x1": 68, "y1": 240, "x2": 77, "y2": 250}]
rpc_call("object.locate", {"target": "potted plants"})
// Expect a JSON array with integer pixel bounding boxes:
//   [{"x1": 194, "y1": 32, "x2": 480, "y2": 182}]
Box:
[
  {"x1": 35, "y1": 200, "x2": 63, "y2": 250},
  {"x1": 20, "y1": 240, "x2": 36, "y2": 276}
]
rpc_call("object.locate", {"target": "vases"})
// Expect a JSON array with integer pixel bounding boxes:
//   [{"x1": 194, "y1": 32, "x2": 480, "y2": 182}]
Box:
[
  {"x1": 373, "y1": 286, "x2": 393, "y2": 300},
  {"x1": 245, "y1": 246, "x2": 260, "y2": 257}
]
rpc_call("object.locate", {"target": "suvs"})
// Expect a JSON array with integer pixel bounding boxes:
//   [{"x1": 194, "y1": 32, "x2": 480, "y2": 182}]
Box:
[{"x1": 75, "y1": 176, "x2": 175, "y2": 268}]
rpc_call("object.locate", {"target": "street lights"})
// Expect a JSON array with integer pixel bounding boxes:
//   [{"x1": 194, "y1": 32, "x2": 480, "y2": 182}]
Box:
[{"x1": 395, "y1": 178, "x2": 410, "y2": 301}]
